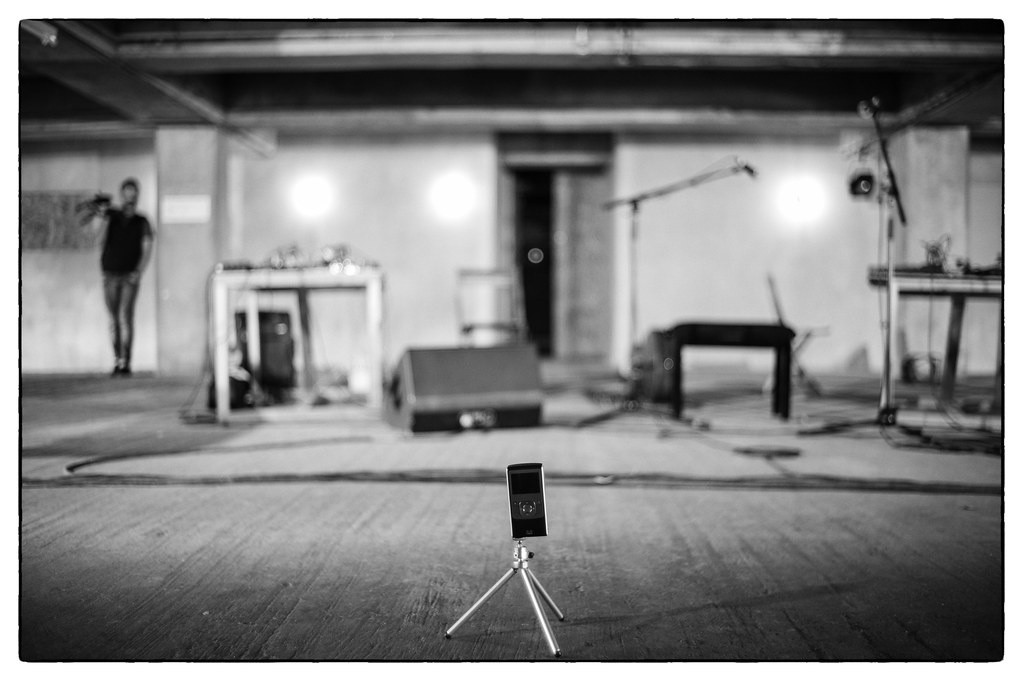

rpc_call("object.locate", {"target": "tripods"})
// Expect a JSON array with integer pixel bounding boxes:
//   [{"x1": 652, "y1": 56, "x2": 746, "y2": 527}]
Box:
[
  {"x1": 575, "y1": 160, "x2": 741, "y2": 430},
  {"x1": 801, "y1": 110, "x2": 943, "y2": 449},
  {"x1": 445, "y1": 540, "x2": 563, "y2": 658}
]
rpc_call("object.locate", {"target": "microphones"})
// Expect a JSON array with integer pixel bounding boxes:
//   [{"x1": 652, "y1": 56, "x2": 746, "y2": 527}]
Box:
[{"x1": 843, "y1": 73, "x2": 884, "y2": 120}]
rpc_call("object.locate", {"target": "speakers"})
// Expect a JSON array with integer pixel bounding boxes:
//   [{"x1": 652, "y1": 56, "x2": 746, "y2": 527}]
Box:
[{"x1": 386, "y1": 346, "x2": 540, "y2": 432}]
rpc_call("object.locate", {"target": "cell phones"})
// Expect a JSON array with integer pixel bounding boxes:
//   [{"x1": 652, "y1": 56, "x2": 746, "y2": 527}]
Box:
[{"x1": 505, "y1": 461, "x2": 547, "y2": 538}]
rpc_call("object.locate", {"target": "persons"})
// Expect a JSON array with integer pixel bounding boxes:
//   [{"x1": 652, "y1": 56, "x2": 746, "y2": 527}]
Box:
[{"x1": 96, "y1": 179, "x2": 153, "y2": 378}]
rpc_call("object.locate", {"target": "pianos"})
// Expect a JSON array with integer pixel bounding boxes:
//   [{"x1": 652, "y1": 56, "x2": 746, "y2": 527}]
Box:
[{"x1": 861, "y1": 266, "x2": 1003, "y2": 425}]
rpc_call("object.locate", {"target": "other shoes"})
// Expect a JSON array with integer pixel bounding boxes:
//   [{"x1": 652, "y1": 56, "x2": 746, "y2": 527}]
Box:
[{"x1": 111, "y1": 366, "x2": 132, "y2": 378}]
